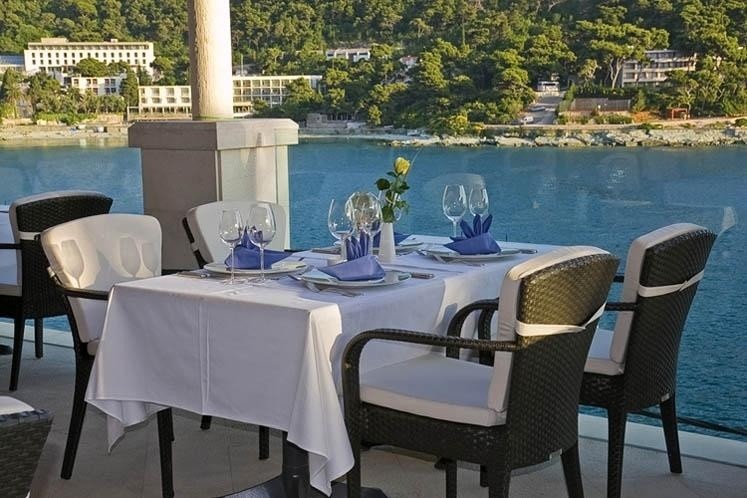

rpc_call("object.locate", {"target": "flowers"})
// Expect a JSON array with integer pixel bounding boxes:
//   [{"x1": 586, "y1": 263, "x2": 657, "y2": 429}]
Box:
[{"x1": 377, "y1": 155, "x2": 412, "y2": 223}]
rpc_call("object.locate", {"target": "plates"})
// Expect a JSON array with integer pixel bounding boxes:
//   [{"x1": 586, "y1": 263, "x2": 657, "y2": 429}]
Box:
[
  {"x1": 204, "y1": 261, "x2": 308, "y2": 274},
  {"x1": 434, "y1": 247, "x2": 522, "y2": 261},
  {"x1": 302, "y1": 268, "x2": 412, "y2": 287},
  {"x1": 372, "y1": 242, "x2": 423, "y2": 255}
]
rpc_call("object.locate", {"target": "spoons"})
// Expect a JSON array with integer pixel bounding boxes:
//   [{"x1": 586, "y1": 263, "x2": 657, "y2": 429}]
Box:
[{"x1": 288, "y1": 274, "x2": 304, "y2": 282}]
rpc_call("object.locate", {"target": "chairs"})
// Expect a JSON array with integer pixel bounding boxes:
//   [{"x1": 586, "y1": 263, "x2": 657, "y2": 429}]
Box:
[
  {"x1": 0, "y1": 395, "x2": 54, "y2": 497},
  {"x1": 478, "y1": 223, "x2": 718, "y2": 497},
  {"x1": 35, "y1": 212, "x2": 271, "y2": 498},
  {"x1": 181, "y1": 198, "x2": 288, "y2": 459},
  {"x1": 1, "y1": 190, "x2": 114, "y2": 392},
  {"x1": 342, "y1": 247, "x2": 621, "y2": 497}
]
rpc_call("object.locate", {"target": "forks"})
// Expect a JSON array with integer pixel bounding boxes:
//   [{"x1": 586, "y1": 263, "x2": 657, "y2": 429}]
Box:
[{"x1": 304, "y1": 281, "x2": 360, "y2": 298}]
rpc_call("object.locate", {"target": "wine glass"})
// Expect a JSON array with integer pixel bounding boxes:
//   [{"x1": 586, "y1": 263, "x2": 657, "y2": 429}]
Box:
[
  {"x1": 468, "y1": 187, "x2": 489, "y2": 217},
  {"x1": 359, "y1": 199, "x2": 385, "y2": 254},
  {"x1": 217, "y1": 208, "x2": 244, "y2": 286},
  {"x1": 327, "y1": 199, "x2": 356, "y2": 260},
  {"x1": 245, "y1": 202, "x2": 276, "y2": 285},
  {"x1": 442, "y1": 184, "x2": 468, "y2": 239}
]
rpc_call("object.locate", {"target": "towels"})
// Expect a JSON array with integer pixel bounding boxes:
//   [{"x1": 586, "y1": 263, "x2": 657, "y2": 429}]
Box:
[
  {"x1": 442, "y1": 213, "x2": 502, "y2": 256},
  {"x1": 371, "y1": 219, "x2": 412, "y2": 247},
  {"x1": 317, "y1": 232, "x2": 386, "y2": 281},
  {"x1": 224, "y1": 225, "x2": 294, "y2": 268}
]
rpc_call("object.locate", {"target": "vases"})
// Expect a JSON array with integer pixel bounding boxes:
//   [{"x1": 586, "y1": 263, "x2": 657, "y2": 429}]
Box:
[{"x1": 376, "y1": 222, "x2": 397, "y2": 265}]
